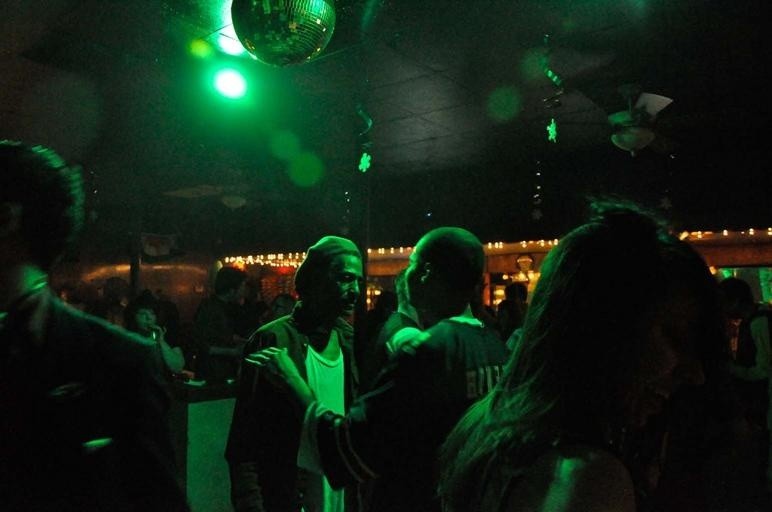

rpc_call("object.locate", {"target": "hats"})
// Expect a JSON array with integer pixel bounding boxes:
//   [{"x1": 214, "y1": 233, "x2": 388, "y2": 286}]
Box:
[{"x1": 295, "y1": 236, "x2": 362, "y2": 297}]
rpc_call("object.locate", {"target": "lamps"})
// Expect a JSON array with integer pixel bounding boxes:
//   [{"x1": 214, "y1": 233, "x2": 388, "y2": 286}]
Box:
[{"x1": 231, "y1": 0, "x2": 336, "y2": 67}]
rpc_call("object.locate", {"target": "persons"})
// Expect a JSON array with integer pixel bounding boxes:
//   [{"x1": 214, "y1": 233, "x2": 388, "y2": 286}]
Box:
[
  {"x1": 356, "y1": 293, "x2": 397, "y2": 370},
  {"x1": 59, "y1": 266, "x2": 296, "y2": 401},
  {"x1": 431, "y1": 195, "x2": 731, "y2": 510},
  {"x1": 508, "y1": 252, "x2": 542, "y2": 295},
  {"x1": 223, "y1": 233, "x2": 369, "y2": 512},
  {"x1": 240, "y1": 224, "x2": 516, "y2": 511},
  {"x1": 470, "y1": 273, "x2": 529, "y2": 364},
  {"x1": 0, "y1": 134, "x2": 199, "y2": 512},
  {"x1": 721, "y1": 278, "x2": 771, "y2": 380}
]
